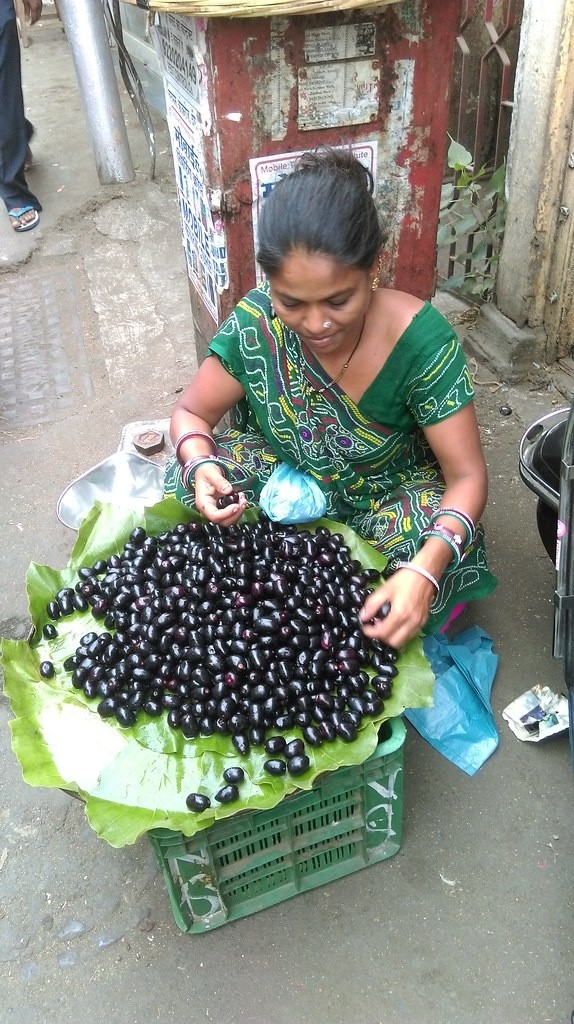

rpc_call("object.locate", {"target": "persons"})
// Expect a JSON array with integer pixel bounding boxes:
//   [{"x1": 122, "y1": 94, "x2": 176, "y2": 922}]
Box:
[
  {"x1": 171, "y1": 144, "x2": 496, "y2": 647},
  {"x1": 0, "y1": 0, "x2": 43, "y2": 232}
]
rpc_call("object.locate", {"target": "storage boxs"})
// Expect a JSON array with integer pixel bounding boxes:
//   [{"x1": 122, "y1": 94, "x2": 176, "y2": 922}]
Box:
[{"x1": 146, "y1": 714, "x2": 407, "y2": 939}]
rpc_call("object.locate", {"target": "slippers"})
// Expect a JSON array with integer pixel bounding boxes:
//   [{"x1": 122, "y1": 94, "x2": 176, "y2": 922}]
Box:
[{"x1": 8, "y1": 205, "x2": 40, "y2": 232}]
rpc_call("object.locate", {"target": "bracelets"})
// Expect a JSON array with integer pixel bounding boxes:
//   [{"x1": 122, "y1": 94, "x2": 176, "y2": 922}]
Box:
[
  {"x1": 395, "y1": 561, "x2": 440, "y2": 593},
  {"x1": 175, "y1": 430, "x2": 231, "y2": 495},
  {"x1": 415, "y1": 506, "x2": 476, "y2": 575}
]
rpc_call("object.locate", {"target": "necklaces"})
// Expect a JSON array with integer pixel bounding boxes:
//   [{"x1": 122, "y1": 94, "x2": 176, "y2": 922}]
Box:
[{"x1": 290, "y1": 312, "x2": 366, "y2": 394}]
